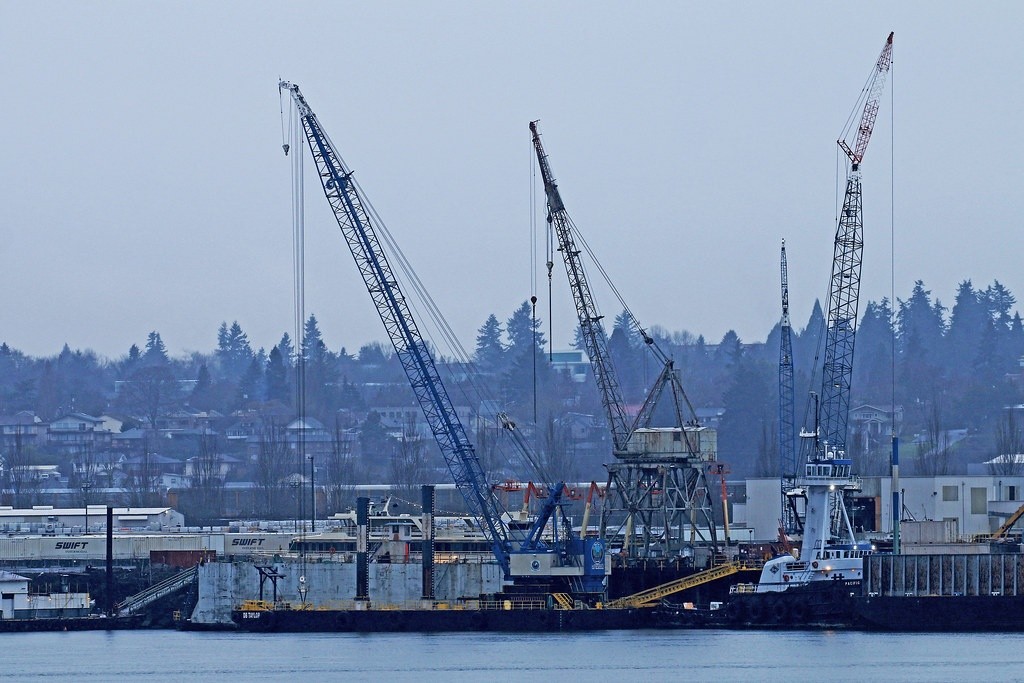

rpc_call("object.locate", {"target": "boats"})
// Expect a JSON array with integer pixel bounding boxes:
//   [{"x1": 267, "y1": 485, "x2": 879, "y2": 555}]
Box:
[
  {"x1": 228, "y1": 605, "x2": 663, "y2": 635},
  {"x1": 0, "y1": 612, "x2": 149, "y2": 634}
]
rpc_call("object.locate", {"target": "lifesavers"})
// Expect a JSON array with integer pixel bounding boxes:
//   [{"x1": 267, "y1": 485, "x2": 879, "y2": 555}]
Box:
[
  {"x1": 388, "y1": 611, "x2": 403, "y2": 627},
  {"x1": 790, "y1": 601, "x2": 809, "y2": 622},
  {"x1": 329, "y1": 547, "x2": 335, "y2": 553},
  {"x1": 772, "y1": 601, "x2": 789, "y2": 622},
  {"x1": 537, "y1": 611, "x2": 552, "y2": 626},
  {"x1": 334, "y1": 611, "x2": 354, "y2": 629},
  {"x1": 471, "y1": 612, "x2": 485, "y2": 627}
]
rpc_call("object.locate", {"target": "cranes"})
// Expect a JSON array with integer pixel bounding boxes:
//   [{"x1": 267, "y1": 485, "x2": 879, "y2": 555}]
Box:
[
  {"x1": 754, "y1": 29, "x2": 898, "y2": 593},
  {"x1": 524, "y1": 116, "x2": 718, "y2": 565},
  {"x1": 275, "y1": 75, "x2": 615, "y2": 609}
]
